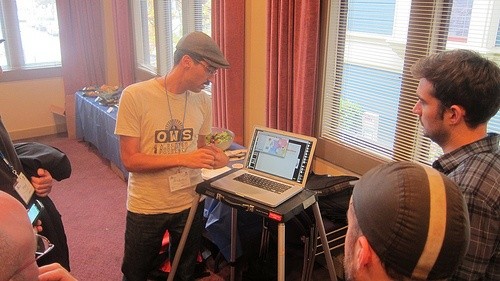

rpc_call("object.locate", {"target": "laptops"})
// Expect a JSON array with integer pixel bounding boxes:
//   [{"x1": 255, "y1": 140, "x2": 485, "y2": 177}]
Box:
[{"x1": 209, "y1": 125, "x2": 317, "y2": 207}]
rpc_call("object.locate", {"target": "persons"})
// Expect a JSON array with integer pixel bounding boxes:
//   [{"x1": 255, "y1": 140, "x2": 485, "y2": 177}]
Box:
[
  {"x1": 408, "y1": 49, "x2": 500, "y2": 281},
  {"x1": 0, "y1": 38, "x2": 79, "y2": 281},
  {"x1": 345, "y1": 161, "x2": 471, "y2": 281},
  {"x1": 114, "y1": 31, "x2": 233, "y2": 281}
]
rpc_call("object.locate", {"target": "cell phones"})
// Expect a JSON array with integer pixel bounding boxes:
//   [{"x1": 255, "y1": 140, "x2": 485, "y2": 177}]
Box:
[{"x1": 27, "y1": 200, "x2": 45, "y2": 226}]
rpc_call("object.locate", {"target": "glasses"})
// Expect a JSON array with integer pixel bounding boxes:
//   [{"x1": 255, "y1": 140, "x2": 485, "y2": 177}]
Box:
[
  {"x1": 8, "y1": 234, "x2": 54, "y2": 281},
  {"x1": 194, "y1": 57, "x2": 217, "y2": 75}
]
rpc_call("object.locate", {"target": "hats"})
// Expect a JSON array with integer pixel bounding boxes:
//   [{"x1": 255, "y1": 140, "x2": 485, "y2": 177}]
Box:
[
  {"x1": 176, "y1": 32, "x2": 231, "y2": 68},
  {"x1": 353, "y1": 160, "x2": 471, "y2": 281}
]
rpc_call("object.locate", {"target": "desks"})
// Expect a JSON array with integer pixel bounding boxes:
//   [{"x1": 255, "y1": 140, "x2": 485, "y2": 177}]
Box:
[{"x1": 74, "y1": 84, "x2": 251, "y2": 263}]
